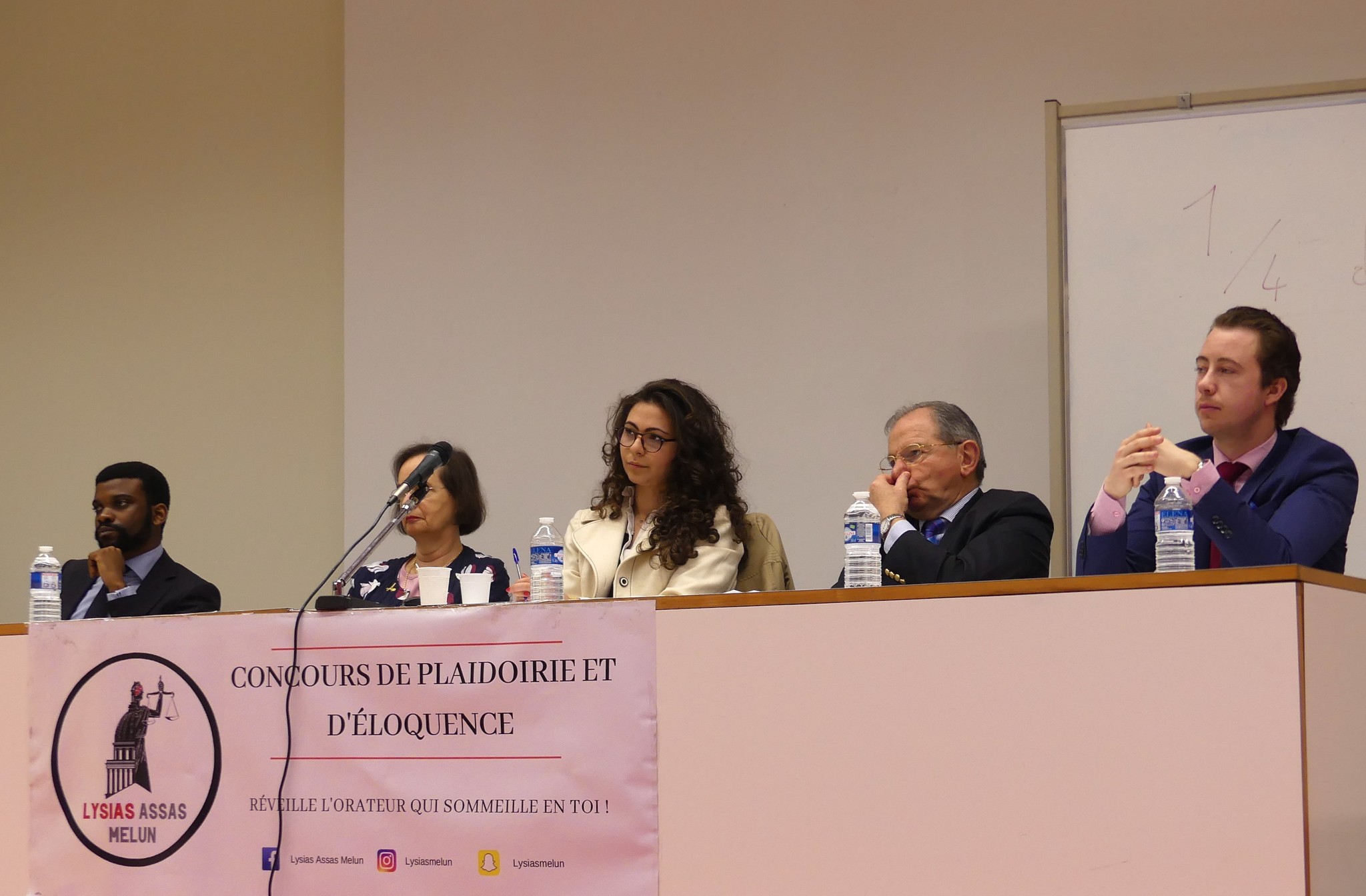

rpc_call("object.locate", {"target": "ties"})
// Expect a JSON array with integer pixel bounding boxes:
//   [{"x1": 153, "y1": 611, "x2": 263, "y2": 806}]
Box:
[
  {"x1": 1210, "y1": 462, "x2": 1249, "y2": 570},
  {"x1": 83, "y1": 564, "x2": 131, "y2": 619},
  {"x1": 921, "y1": 518, "x2": 946, "y2": 544}
]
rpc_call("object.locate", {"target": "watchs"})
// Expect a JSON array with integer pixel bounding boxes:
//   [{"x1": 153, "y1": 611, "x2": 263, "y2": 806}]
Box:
[
  {"x1": 880, "y1": 513, "x2": 907, "y2": 542},
  {"x1": 1193, "y1": 458, "x2": 1206, "y2": 472}
]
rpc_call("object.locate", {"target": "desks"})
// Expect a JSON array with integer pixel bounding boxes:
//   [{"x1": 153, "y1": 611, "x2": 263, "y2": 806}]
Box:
[{"x1": 0, "y1": 564, "x2": 1366, "y2": 896}]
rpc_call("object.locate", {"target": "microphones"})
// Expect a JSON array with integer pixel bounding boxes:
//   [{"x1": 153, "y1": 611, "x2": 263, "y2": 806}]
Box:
[{"x1": 386, "y1": 440, "x2": 453, "y2": 506}]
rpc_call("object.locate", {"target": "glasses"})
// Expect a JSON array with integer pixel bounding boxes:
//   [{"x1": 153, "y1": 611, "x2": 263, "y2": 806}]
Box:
[
  {"x1": 879, "y1": 443, "x2": 961, "y2": 474},
  {"x1": 615, "y1": 427, "x2": 678, "y2": 453}
]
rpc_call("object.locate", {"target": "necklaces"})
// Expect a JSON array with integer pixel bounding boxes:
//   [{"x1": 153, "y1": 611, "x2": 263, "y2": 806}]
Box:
[
  {"x1": 638, "y1": 518, "x2": 645, "y2": 523},
  {"x1": 404, "y1": 547, "x2": 462, "y2": 590}
]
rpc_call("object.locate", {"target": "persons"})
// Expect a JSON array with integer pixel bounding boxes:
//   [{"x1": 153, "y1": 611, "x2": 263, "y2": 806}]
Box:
[
  {"x1": 506, "y1": 377, "x2": 752, "y2": 603},
  {"x1": 347, "y1": 443, "x2": 510, "y2": 608},
  {"x1": 60, "y1": 461, "x2": 220, "y2": 621},
  {"x1": 1076, "y1": 306, "x2": 1359, "y2": 575},
  {"x1": 830, "y1": 402, "x2": 1054, "y2": 589}
]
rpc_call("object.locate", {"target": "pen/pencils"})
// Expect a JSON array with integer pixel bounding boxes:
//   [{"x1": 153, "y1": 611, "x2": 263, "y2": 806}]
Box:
[{"x1": 513, "y1": 547, "x2": 527, "y2": 602}]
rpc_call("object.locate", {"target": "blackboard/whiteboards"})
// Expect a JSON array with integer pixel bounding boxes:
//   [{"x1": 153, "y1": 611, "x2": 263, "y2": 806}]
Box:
[{"x1": 1043, "y1": 76, "x2": 1366, "y2": 581}]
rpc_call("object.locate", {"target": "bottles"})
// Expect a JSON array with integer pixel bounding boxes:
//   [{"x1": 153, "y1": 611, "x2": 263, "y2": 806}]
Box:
[
  {"x1": 29, "y1": 546, "x2": 61, "y2": 622},
  {"x1": 1153, "y1": 476, "x2": 1195, "y2": 572},
  {"x1": 530, "y1": 517, "x2": 564, "y2": 603},
  {"x1": 843, "y1": 490, "x2": 883, "y2": 588}
]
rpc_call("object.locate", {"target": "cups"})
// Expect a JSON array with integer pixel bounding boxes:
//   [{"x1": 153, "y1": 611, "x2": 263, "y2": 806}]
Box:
[
  {"x1": 416, "y1": 567, "x2": 452, "y2": 605},
  {"x1": 458, "y1": 574, "x2": 494, "y2": 605}
]
